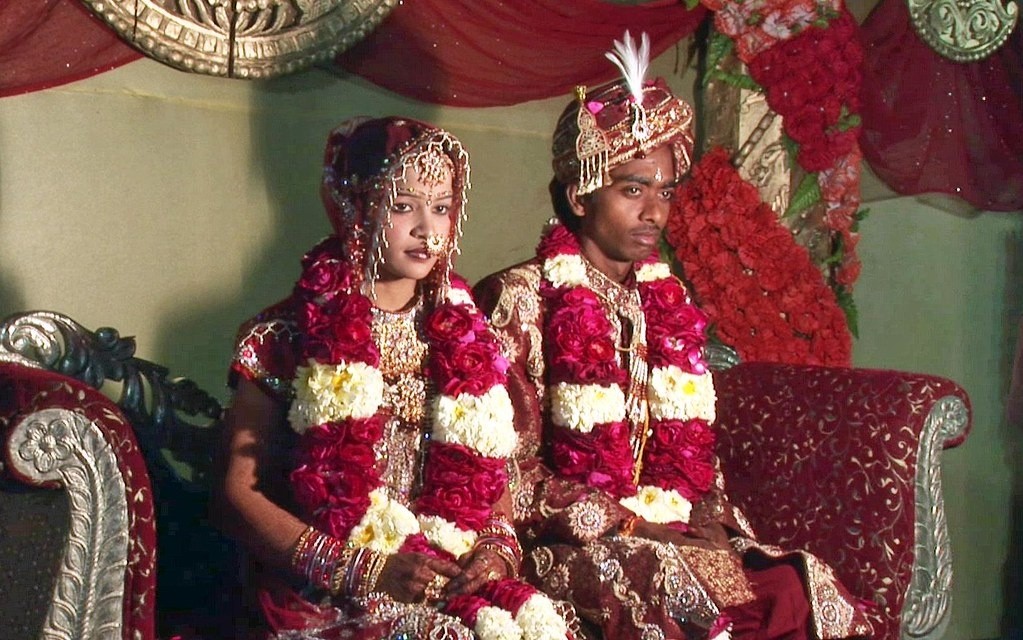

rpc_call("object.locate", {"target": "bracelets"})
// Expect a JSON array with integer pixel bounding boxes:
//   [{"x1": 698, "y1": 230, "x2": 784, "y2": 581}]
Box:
[
  {"x1": 288, "y1": 525, "x2": 388, "y2": 610},
  {"x1": 473, "y1": 512, "x2": 523, "y2": 581}
]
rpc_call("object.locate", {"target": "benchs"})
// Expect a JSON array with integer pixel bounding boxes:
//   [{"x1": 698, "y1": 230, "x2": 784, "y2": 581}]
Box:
[{"x1": 0, "y1": 308, "x2": 971, "y2": 640}]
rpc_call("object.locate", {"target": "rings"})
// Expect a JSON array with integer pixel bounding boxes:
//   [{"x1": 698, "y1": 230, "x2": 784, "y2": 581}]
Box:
[
  {"x1": 475, "y1": 556, "x2": 488, "y2": 565},
  {"x1": 424, "y1": 584, "x2": 441, "y2": 601},
  {"x1": 429, "y1": 573, "x2": 444, "y2": 589},
  {"x1": 486, "y1": 568, "x2": 502, "y2": 580}
]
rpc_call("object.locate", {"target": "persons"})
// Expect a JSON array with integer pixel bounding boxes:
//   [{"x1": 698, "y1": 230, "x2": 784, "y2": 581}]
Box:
[
  {"x1": 223, "y1": 114, "x2": 569, "y2": 640},
  {"x1": 477, "y1": 77, "x2": 880, "y2": 640}
]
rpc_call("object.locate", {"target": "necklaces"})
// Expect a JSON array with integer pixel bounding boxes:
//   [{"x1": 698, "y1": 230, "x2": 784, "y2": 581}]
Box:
[
  {"x1": 366, "y1": 282, "x2": 428, "y2": 499},
  {"x1": 582, "y1": 257, "x2": 651, "y2": 485}
]
